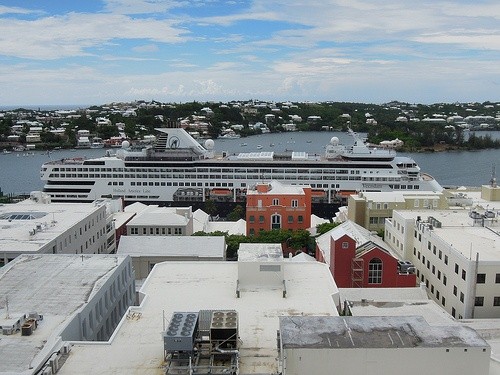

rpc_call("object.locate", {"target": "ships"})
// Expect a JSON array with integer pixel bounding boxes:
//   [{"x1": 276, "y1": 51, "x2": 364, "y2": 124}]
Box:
[{"x1": 39, "y1": 127, "x2": 449, "y2": 217}]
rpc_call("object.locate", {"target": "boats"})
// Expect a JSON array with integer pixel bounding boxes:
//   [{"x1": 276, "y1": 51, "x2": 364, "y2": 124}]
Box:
[
  {"x1": 1, "y1": 148, "x2": 13, "y2": 155},
  {"x1": 13, "y1": 145, "x2": 29, "y2": 152},
  {"x1": 90, "y1": 142, "x2": 104, "y2": 149},
  {"x1": 331, "y1": 127, "x2": 396, "y2": 161},
  {"x1": 240, "y1": 136, "x2": 313, "y2": 152},
  {"x1": 110, "y1": 142, "x2": 122, "y2": 148}
]
